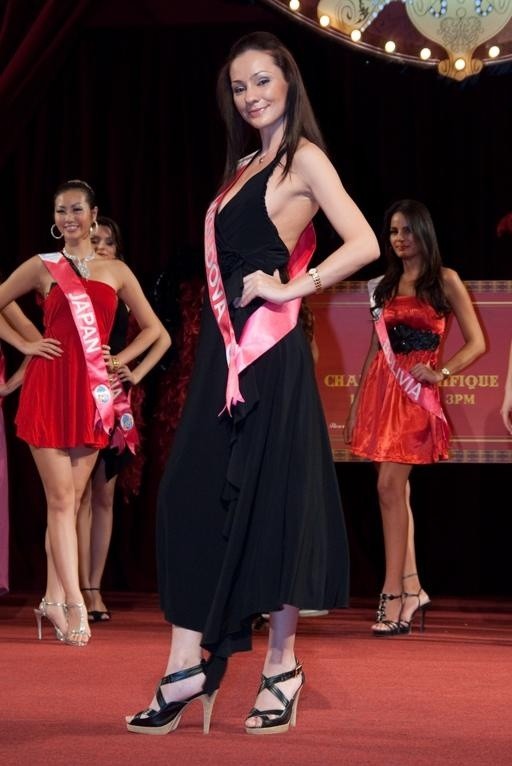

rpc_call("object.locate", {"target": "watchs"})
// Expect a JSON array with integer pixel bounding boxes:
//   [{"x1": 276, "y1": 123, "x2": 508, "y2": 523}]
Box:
[
  {"x1": 111, "y1": 355, "x2": 119, "y2": 372},
  {"x1": 436, "y1": 367, "x2": 450, "y2": 381},
  {"x1": 309, "y1": 268, "x2": 323, "y2": 293}
]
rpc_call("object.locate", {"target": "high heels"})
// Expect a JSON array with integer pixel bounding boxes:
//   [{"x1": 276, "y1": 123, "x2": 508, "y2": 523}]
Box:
[
  {"x1": 244, "y1": 655, "x2": 306, "y2": 735},
  {"x1": 371, "y1": 592, "x2": 404, "y2": 635},
  {"x1": 124, "y1": 658, "x2": 220, "y2": 736},
  {"x1": 399, "y1": 587, "x2": 432, "y2": 635},
  {"x1": 64, "y1": 600, "x2": 92, "y2": 649},
  {"x1": 33, "y1": 597, "x2": 68, "y2": 641}
]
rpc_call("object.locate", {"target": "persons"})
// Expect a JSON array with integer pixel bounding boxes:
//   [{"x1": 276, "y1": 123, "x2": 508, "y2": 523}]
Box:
[
  {"x1": 1, "y1": 213, "x2": 172, "y2": 622},
  {"x1": 123, "y1": 32, "x2": 382, "y2": 735},
  {"x1": 1, "y1": 176, "x2": 162, "y2": 646},
  {"x1": 341, "y1": 192, "x2": 487, "y2": 637},
  {"x1": 498, "y1": 343, "x2": 512, "y2": 433}
]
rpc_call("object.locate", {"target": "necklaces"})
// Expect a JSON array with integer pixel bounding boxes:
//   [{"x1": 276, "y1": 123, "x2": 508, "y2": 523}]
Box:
[
  {"x1": 62, "y1": 248, "x2": 96, "y2": 281},
  {"x1": 256, "y1": 137, "x2": 286, "y2": 164}
]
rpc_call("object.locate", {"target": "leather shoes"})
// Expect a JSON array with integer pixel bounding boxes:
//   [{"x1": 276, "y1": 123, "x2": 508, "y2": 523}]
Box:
[
  {"x1": 80, "y1": 588, "x2": 97, "y2": 623},
  {"x1": 90, "y1": 588, "x2": 112, "y2": 623}
]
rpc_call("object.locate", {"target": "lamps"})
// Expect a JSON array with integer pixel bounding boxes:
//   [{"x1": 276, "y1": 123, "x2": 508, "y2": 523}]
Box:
[{"x1": 270, "y1": 0, "x2": 511, "y2": 81}]
